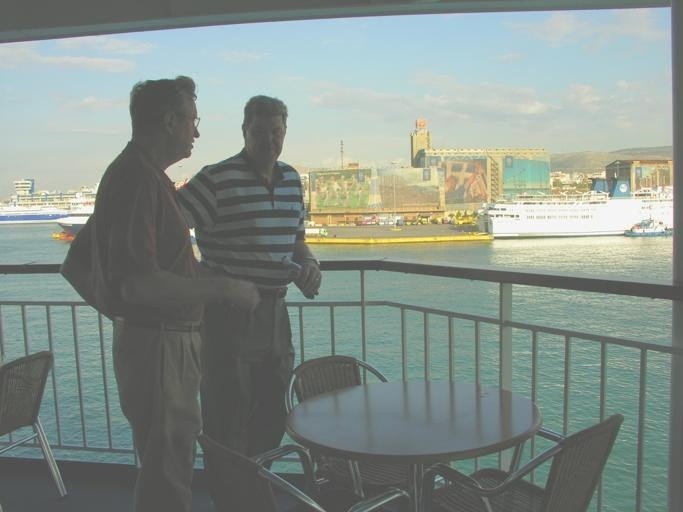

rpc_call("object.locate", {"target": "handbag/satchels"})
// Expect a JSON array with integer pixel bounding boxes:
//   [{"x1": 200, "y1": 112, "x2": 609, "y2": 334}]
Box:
[{"x1": 57, "y1": 213, "x2": 122, "y2": 321}]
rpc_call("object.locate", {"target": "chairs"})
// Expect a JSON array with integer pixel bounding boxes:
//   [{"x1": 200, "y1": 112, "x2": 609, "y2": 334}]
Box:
[
  {"x1": 0, "y1": 350, "x2": 69, "y2": 501},
  {"x1": 194, "y1": 430, "x2": 415, "y2": 512},
  {"x1": 287, "y1": 354, "x2": 414, "y2": 494},
  {"x1": 423, "y1": 412, "x2": 627, "y2": 511}
]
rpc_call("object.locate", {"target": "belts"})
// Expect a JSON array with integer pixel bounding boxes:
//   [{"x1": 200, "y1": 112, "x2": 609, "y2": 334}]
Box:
[
  {"x1": 256, "y1": 284, "x2": 288, "y2": 300},
  {"x1": 113, "y1": 315, "x2": 204, "y2": 333}
]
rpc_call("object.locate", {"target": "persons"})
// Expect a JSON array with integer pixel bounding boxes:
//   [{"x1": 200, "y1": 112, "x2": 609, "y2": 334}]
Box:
[
  {"x1": 60, "y1": 77, "x2": 205, "y2": 511},
  {"x1": 174, "y1": 96, "x2": 322, "y2": 511}
]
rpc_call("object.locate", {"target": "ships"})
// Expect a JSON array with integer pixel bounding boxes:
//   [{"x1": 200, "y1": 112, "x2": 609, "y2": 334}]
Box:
[
  {"x1": 477, "y1": 176, "x2": 674, "y2": 239},
  {"x1": 55, "y1": 215, "x2": 90, "y2": 237},
  {"x1": 0, "y1": 205, "x2": 65, "y2": 223},
  {"x1": 304, "y1": 214, "x2": 331, "y2": 236},
  {"x1": 0, "y1": 186, "x2": 99, "y2": 218},
  {"x1": 475, "y1": 185, "x2": 673, "y2": 237}
]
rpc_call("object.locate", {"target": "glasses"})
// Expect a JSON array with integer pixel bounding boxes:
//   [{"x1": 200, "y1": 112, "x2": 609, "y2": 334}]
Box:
[{"x1": 179, "y1": 112, "x2": 201, "y2": 129}]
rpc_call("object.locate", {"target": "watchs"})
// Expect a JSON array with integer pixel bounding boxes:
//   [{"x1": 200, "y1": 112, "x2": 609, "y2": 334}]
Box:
[{"x1": 302, "y1": 255, "x2": 321, "y2": 266}]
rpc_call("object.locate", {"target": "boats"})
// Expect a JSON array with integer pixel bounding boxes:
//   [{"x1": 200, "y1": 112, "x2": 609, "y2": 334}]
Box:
[
  {"x1": 624, "y1": 218, "x2": 667, "y2": 236},
  {"x1": 623, "y1": 217, "x2": 673, "y2": 236},
  {"x1": 55, "y1": 217, "x2": 96, "y2": 235}
]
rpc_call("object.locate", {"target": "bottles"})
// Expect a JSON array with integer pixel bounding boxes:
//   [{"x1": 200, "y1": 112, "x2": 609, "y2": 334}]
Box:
[{"x1": 280, "y1": 256, "x2": 319, "y2": 300}]
